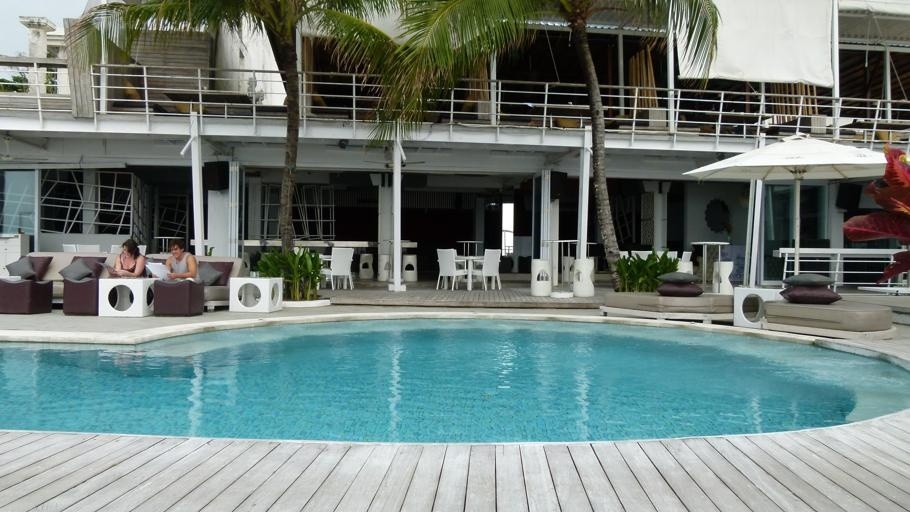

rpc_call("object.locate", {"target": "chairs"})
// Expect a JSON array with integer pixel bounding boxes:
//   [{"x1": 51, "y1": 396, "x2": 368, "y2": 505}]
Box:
[{"x1": 310, "y1": 245, "x2": 504, "y2": 294}]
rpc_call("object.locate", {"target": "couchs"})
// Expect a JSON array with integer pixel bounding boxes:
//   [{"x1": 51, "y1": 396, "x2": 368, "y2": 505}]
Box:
[
  {"x1": 829, "y1": 294, "x2": 910, "y2": 331},
  {"x1": 599, "y1": 290, "x2": 761, "y2": 326},
  {"x1": 1, "y1": 248, "x2": 243, "y2": 318},
  {"x1": 756, "y1": 297, "x2": 900, "y2": 343}
]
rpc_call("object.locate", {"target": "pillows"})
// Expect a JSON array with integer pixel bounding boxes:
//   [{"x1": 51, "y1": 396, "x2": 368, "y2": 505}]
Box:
[
  {"x1": 777, "y1": 286, "x2": 845, "y2": 306},
  {"x1": 782, "y1": 272, "x2": 837, "y2": 286},
  {"x1": 655, "y1": 281, "x2": 705, "y2": 298},
  {"x1": 657, "y1": 270, "x2": 704, "y2": 283}
]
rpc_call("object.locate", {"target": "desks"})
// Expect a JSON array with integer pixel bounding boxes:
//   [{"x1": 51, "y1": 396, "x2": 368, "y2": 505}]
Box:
[
  {"x1": 227, "y1": 274, "x2": 286, "y2": 315},
  {"x1": 456, "y1": 237, "x2": 486, "y2": 278},
  {"x1": 544, "y1": 238, "x2": 581, "y2": 299},
  {"x1": 687, "y1": 239, "x2": 731, "y2": 294}
]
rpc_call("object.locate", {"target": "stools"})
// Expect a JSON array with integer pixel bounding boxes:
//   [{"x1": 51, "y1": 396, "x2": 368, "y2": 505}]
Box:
[
  {"x1": 561, "y1": 254, "x2": 576, "y2": 285},
  {"x1": 709, "y1": 259, "x2": 738, "y2": 297},
  {"x1": 571, "y1": 257, "x2": 596, "y2": 299},
  {"x1": 530, "y1": 258, "x2": 555, "y2": 300}
]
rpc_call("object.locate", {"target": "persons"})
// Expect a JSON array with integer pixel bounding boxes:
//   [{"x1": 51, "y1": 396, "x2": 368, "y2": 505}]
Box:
[
  {"x1": 105, "y1": 240, "x2": 146, "y2": 279},
  {"x1": 152, "y1": 240, "x2": 203, "y2": 284}
]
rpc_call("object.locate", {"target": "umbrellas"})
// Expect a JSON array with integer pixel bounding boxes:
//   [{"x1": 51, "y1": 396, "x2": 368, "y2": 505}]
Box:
[{"x1": 681, "y1": 132, "x2": 909, "y2": 276}]
